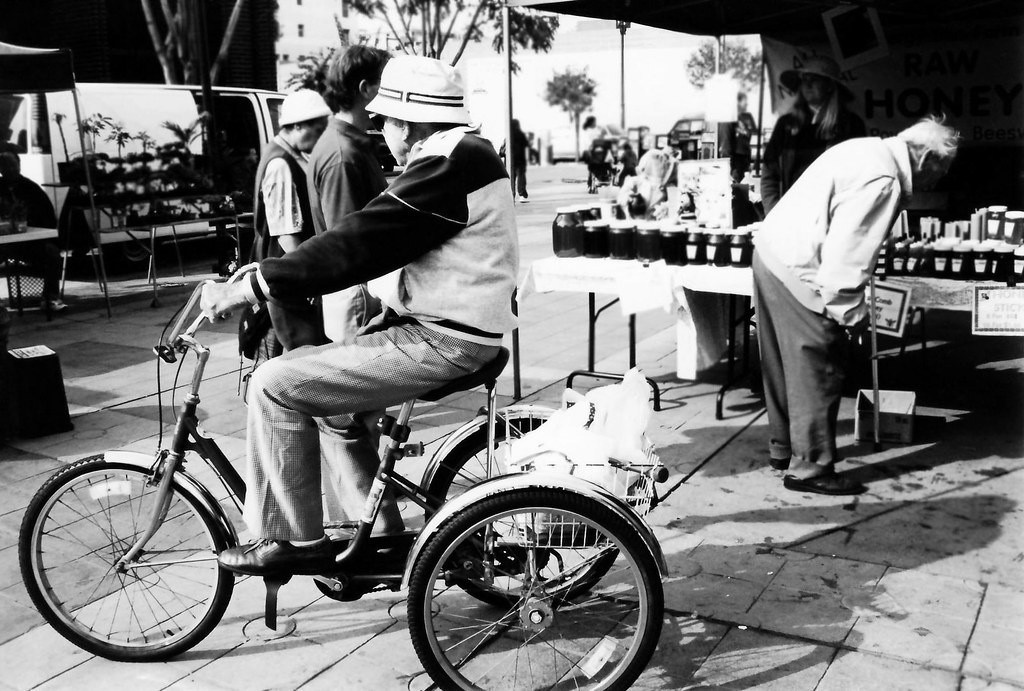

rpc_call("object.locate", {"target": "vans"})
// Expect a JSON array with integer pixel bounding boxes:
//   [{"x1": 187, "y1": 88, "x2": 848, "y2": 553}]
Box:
[{"x1": 0, "y1": 83, "x2": 289, "y2": 275}]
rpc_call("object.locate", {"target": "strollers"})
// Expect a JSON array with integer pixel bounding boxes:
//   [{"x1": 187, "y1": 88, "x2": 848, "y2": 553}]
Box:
[{"x1": 580, "y1": 140, "x2": 624, "y2": 194}]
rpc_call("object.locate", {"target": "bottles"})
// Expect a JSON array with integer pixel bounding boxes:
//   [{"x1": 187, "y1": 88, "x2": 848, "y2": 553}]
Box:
[{"x1": 551, "y1": 188, "x2": 1024, "y2": 285}]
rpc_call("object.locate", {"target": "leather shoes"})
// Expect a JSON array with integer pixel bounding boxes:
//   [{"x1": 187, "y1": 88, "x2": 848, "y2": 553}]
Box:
[
  {"x1": 770, "y1": 457, "x2": 791, "y2": 470},
  {"x1": 784, "y1": 471, "x2": 863, "y2": 495},
  {"x1": 217, "y1": 534, "x2": 337, "y2": 576}
]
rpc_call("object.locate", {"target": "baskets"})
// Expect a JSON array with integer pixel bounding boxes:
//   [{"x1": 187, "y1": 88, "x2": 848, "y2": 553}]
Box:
[{"x1": 504, "y1": 408, "x2": 665, "y2": 550}]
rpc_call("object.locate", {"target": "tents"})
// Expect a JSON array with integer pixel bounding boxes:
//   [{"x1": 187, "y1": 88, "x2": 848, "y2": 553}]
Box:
[{"x1": 0, "y1": 44, "x2": 113, "y2": 321}]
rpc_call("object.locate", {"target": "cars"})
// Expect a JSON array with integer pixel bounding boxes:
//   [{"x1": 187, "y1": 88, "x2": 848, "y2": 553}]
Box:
[{"x1": 528, "y1": 117, "x2": 622, "y2": 166}]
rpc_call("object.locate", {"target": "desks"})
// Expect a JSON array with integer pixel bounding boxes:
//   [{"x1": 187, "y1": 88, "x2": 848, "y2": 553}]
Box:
[
  {"x1": 102, "y1": 212, "x2": 254, "y2": 310},
  {"x1": 532, "y1": 254, "x2": 1024, "y2": 421},
  {"x1": 0, "y1": 227, "x2": 60, "y2": 324}
]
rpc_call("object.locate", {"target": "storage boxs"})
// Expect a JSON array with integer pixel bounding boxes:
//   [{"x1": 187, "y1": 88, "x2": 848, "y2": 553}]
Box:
[{"x1": 855, "y1": 389, "x2": 916, "y2": 443}]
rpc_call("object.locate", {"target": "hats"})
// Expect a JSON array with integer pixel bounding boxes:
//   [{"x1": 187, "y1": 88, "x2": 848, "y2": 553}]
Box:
[
  {"x1": 278, "y1": 88, "x2": 332, "y2": 128},
  {"x1": 364, "y1": 55, "x2": 473, "y2": 125},
  {"x1": 779, "y1": 54, "x2": 857, "y2": 103}
]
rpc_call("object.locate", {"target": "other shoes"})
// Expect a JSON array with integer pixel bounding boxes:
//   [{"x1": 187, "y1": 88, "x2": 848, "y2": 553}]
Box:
[{"x1": 520, "y1": 196, "x2": 530, "y2": 202}]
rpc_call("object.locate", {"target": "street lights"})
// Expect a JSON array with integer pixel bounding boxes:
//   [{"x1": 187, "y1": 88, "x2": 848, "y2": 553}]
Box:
[{"x1": 616, "y1": 20, "x2": 631, "y2": 129}]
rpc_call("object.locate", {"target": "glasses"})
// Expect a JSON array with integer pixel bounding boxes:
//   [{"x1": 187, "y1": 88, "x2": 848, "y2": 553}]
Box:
[{"x1": 369, "y1": 112, "x2": 384, "y2": 132}]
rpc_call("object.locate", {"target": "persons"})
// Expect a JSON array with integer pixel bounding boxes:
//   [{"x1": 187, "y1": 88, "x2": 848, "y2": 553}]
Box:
[
  {"x1": 761, "y1": 55, "x2": 866, "y2": 215},
  {"x1": 723, "y1": 92, "x2": 758, "y2": 182},
  {"x1": 752, "y1": 114, "x2": 961, "y2": 494},
  {"x1": 504, "y1": 118, "x2": 639, "y2": 203},
  {"x1": 306, "y1": 46, "x2": 395, "y2": 536},
  {"x1": 200, "y1": 54, "x2": 518, "y2": 575},
  {"x1": 0, "y1": 148, "x2": 67, "y2": 312},
  {"x1": 252, "y1": 87, "x2": 332, "y2": 348}
]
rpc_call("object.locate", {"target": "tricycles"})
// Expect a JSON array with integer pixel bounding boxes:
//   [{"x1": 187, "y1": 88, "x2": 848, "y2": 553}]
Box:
[{"x1": 18, "y1": 262, "x2": 668, "y2": 691}]
